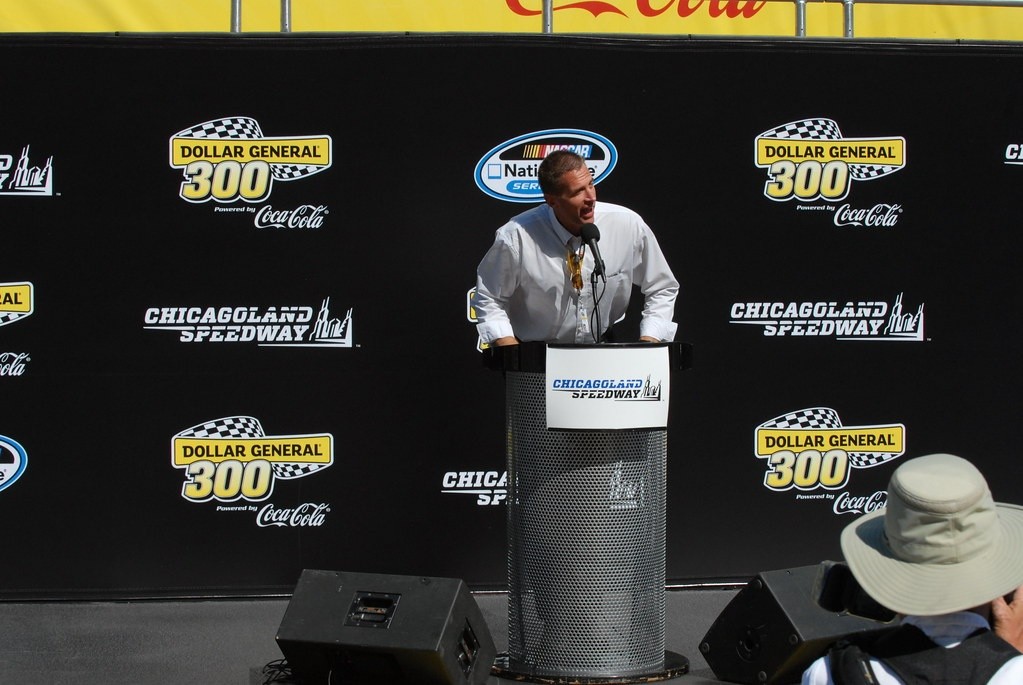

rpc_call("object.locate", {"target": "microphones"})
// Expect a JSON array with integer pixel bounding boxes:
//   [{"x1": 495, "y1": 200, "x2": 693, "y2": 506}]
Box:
[{"x1": 581, "y1": 222, "x2": 607, "y2": 284}]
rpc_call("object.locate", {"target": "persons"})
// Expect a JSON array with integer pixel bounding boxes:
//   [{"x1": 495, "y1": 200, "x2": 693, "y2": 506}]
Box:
[
  {"x1": 801, "y1": 453, "x2": 1023, "y2": 685},
  {"x1": 470, "y1": 150, "x2": 679, "y2": 348}
]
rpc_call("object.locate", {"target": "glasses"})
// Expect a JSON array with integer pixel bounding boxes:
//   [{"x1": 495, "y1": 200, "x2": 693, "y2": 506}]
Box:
[{"x1": 570, "y1": 254, "x2": 583, "y2": 295}]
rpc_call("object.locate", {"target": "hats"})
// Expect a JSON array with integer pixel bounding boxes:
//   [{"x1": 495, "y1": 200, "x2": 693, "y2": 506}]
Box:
[{"x1": 840, "y1": 452, "x2": 1022, "y2": 617}]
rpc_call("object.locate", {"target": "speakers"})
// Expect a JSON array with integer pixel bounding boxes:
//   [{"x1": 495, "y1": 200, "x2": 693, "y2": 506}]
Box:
[
  {"x1": 698, "y1": 559, "x2": 900, "y2": 685},
  {"x1": 276, "y1": 569, "x2": 497, "y2": 685}
]
